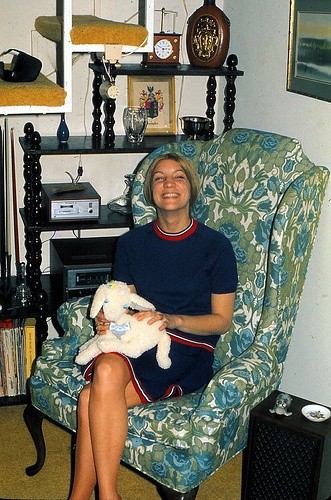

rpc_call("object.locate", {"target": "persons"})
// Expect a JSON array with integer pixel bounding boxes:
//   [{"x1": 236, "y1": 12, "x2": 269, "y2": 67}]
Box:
[{"x1": 64, "y1": 151, "x2": 241, "y2": 500}]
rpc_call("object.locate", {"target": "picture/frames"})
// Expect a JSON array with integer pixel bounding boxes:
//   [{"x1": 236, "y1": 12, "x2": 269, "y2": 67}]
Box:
[
  {"x1": 286, "y1": 0, "x2": 331, "y2": 103},
  {"x1": 128, "y1": 75, "x2": 176, "y2": 136}
]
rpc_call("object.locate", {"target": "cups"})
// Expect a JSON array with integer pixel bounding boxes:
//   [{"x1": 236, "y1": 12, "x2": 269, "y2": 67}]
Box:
[{"x1": 123, "y1": 106, "x2": 149, "y2": 144}]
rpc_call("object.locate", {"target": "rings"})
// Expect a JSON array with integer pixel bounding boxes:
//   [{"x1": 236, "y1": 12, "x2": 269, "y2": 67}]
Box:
[{"x1": 158, "y1": 314, "x2": 163, "y2": 320}]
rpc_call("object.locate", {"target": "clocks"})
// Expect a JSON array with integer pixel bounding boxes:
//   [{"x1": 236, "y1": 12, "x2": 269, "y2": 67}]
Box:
[{"x1": 152, "y1": 34, "x2": 181, "y2": 63}]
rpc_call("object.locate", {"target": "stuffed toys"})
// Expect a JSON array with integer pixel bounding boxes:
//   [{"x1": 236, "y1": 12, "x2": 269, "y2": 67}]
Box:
[{"x1": 76, "y1": 277, "x2": 174, "y2": 371}]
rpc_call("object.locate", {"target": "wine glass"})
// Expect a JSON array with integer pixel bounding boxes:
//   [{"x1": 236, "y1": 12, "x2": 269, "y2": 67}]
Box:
[{"x1": 179, "y1": 116, "x2": 210, "y2": 142}]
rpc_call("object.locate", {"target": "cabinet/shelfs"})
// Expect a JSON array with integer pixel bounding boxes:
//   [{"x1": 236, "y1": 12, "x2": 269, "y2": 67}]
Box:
[
  {"x1": 0, "y1": 0, "x2": 155, "y2": 115},
  {"x1": 0, "y1": 52, "x2": 244, "y2": 407}
]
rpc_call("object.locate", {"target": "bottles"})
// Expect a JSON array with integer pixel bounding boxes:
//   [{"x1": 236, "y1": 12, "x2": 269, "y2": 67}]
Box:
[{"x1": 57, "y1": 113, "x2": 69, "y2": 143}]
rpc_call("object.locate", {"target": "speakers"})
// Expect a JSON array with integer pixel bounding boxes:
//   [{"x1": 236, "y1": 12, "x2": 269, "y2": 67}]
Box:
[{"x1": 243, "y1": 389, "x2": 331, "y2": 500}]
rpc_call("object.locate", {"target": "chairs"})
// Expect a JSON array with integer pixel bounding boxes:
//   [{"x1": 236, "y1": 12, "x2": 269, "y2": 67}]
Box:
[{"x1": 22, "y1": 127, "x2": 330, "y2": 500}]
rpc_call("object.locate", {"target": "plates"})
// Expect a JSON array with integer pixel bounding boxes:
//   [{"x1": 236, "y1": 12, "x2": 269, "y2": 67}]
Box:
[{"x1": 301, "y1": 404, "x2": 331, "y2": 422}]
